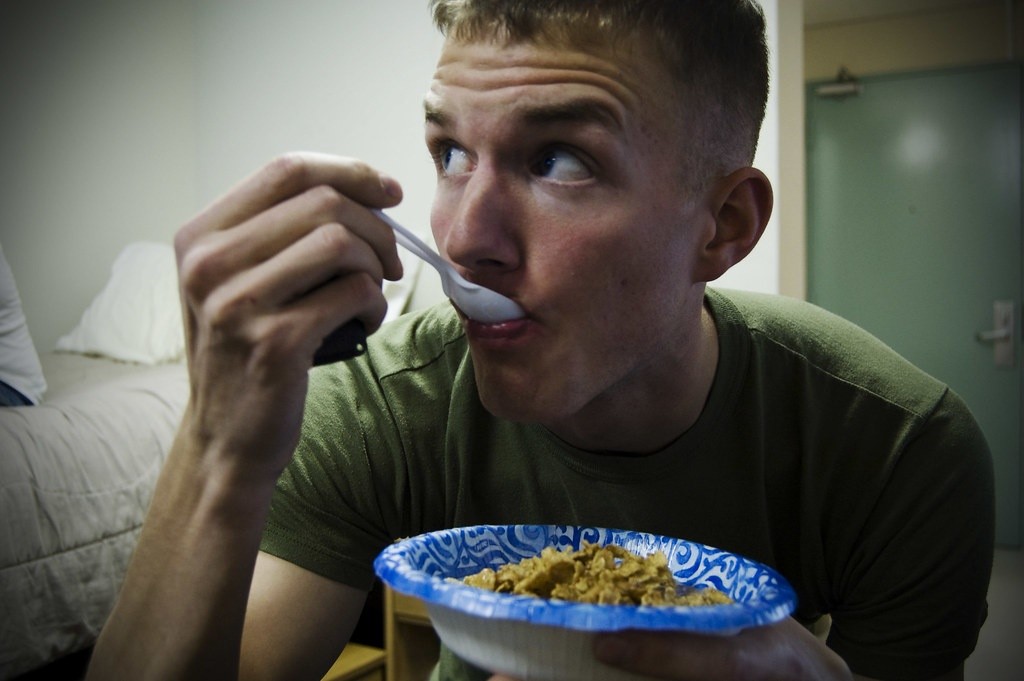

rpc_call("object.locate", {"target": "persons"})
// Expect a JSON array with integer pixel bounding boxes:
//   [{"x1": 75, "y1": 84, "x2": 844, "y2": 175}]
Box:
[
  {"x1": 0, "y1": 255, "x2": 49, "y2": 407},
  {"x1": 88, "y1": 1, "x2": 993, "y2": 681}
]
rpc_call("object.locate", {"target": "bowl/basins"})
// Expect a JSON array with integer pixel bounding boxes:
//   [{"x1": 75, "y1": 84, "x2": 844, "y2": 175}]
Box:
[{"x1": 372, "y1": 524, "x2": 798, "y2": 681}]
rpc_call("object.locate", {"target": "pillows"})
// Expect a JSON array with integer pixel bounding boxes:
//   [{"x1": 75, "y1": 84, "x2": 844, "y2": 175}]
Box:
[{"x1": 53, "y1": 240, "x2": 184, "y2": 365}]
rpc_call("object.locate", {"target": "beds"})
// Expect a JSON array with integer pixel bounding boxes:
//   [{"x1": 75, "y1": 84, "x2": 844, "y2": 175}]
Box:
[{"x1": 0, "y1": 349, "x2": 192, "y2": 681}]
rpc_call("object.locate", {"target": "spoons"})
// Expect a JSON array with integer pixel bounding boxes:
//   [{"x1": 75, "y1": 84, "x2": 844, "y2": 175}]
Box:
[{"x1": 368, "y1": 208, "x2": 527, "y2": 323}]
